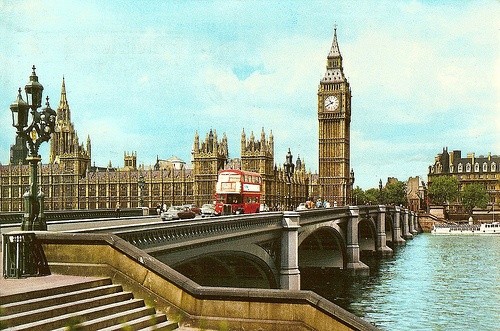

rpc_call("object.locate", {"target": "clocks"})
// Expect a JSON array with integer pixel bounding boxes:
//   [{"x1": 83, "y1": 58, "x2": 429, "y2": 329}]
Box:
[{"x1": 323, "y1": 95, "x2": 341, "y2": 113}]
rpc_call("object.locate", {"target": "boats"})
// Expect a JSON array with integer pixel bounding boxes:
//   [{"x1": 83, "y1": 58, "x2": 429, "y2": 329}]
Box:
[{"x1": 431, "y1": 221, "x2": 500, "y2": 235}]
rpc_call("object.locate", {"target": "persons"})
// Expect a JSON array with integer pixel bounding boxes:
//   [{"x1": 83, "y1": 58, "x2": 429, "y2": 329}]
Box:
[{"x1": 115, "y1": 202, "x2": 121, "y2": 218}]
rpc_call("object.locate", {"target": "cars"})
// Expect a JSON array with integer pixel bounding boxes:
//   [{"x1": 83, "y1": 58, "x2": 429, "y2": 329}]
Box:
[
  {"x1": 296, "y1": 202, "x2": 308, "y2": 211},
  {"x1": 260, "y1": 203, "x2": 270, "y2": 212},
  {"x1": 160, "y1": 203, "x2": 215, "y2": 221}
]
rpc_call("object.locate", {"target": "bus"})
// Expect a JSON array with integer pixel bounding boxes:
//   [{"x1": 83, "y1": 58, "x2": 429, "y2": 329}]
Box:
[{"x1": 213, "y1": 169, "x2": 263, "y2": 217}]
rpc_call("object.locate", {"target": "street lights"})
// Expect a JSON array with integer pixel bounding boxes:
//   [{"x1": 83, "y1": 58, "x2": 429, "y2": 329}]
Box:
[
  {"x1": 283, "y1": 147, "x2": 295, "y2": 211},
  {"x1": 379, "y1": 178, "x2": 383, "y2": 205},
  {"x1": 8, "y1": 64, "x2": 57, "y2": 231},
  {"x1": 349, "y1": 168, "x2": 355, "y2": 206}
]
